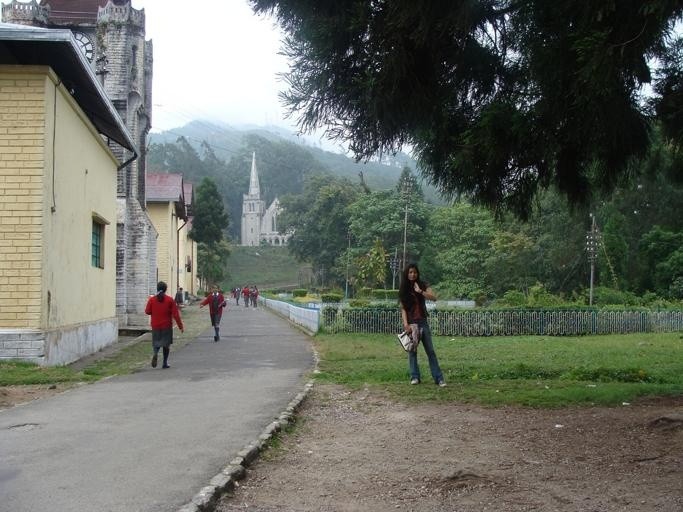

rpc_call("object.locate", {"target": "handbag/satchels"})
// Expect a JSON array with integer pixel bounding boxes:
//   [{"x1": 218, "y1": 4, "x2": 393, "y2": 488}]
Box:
[{"x1": 398, "y1": 330, "x2": 413, "y2": 351}]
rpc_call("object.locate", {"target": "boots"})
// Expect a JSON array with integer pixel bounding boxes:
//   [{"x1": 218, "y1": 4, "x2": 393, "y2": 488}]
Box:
[
  {"x1": 162, "y1": 347, "x2": 170, "y2": 368},
  {"x1": 214, "y1": 327, "x2": 220, "y2": 341},
  {"x1": 152, "y1": 347, "x2": 159, "y2": 368}
]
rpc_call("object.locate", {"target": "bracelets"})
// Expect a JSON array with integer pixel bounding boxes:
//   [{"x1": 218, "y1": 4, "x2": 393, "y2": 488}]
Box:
[{"x1": 420, "y1": 289, "x2": 423, "y2": 294}]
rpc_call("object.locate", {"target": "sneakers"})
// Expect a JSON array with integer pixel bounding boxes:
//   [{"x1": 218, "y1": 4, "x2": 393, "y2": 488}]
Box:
[
  {"x1": 411, "y1": 378, "x2": 419, "y2": 385},
  {"x1": 439, "y1": 381, "x2": 446, "y2": 387}
]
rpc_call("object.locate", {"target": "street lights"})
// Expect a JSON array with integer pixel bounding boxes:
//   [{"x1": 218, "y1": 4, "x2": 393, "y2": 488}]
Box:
[
  {"x1": 345, "y1": 229, "x2": 355, "y2": 298},
  {"x1": 388, "y1": 249, "x2": 401, "y2": 292},
  {"x1": 584, "y1": 215, "x2": 602, "y2": 306},
  {"x1": 397, "y1": 172, "x2": 415, "y2": 274}
]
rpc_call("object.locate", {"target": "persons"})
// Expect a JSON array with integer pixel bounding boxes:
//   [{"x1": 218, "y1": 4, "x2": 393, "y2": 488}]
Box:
[
  {"x1": 230, "y1": 286, "x2": 259, "y2": 309},
  {"x1": 397, "y1": 263, "x2": 447, "y2": 387},
  {"x1": 175, "y1": 287, "x2": 183, "y2": 311},
  {"x1": 199, "y1": 284, "x2": 228, "y2": 342},
  {"x1": 145, "y1": 281, "x2": 184, "y2": 369}
]
